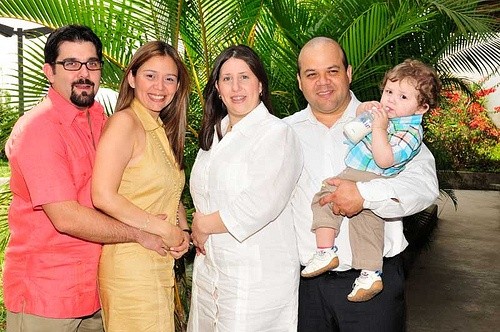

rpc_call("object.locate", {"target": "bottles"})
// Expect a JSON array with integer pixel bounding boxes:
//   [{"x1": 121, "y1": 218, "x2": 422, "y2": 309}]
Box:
[{"x1": 342, "y1": 104, "x2": 390, "y2": 144}]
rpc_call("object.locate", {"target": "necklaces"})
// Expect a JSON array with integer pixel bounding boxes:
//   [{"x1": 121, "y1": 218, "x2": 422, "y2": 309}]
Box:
[
  {"x1": 150, "y1": 128, "x2": 183, "y2": 227},
  {"x1": 229, "y1": 121, "x2": 232, "y2": 128}
]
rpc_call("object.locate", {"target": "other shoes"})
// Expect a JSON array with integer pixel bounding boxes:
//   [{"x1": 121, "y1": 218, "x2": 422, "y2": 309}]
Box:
[
  {"x1": 301, "y1": 246, "x2": 339, "y2": 277},
  {"x1": 347, "y1": 270, "x2": 383, "y2": 302}
]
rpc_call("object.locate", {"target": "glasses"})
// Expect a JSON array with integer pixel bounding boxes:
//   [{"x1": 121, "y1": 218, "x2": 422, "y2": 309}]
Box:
[{"x1": 48, "y1": 60, "x2": 104, "y2": 71}]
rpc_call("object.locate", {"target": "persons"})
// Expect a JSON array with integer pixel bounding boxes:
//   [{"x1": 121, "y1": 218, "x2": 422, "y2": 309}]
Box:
[
  {"x1": 300, "y1": 59, "x2": 440, "y2": 302},
  {"x1": 91, "y1": 42, "x2": 190, "y2": 332},
  {"x1": 184, "y1": 45, "x2": 305, "y2": 332},
  {"x1": 3, "y1": 24, "x2": 170, "y2": 332},
  {"x1": 282, "y1": 37, "x2": 440, "y2": 332}
]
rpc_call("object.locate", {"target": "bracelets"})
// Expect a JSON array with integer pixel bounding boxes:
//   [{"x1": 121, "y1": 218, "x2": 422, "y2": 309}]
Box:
[
  {"x1": 183, "y1": 229, "x2": 194, "y2": 249},
  {"x1": 140, "y1": 212, "x2": 151, "y2": 230}
]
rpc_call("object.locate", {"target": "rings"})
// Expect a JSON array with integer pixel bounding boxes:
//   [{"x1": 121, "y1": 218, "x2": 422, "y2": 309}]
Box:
[{"x1": 340, "y1": 210, "x2": 347, "y2": 215}]
